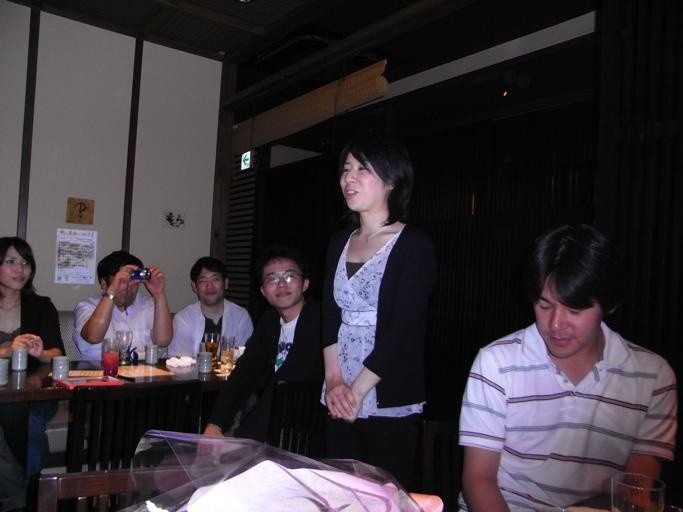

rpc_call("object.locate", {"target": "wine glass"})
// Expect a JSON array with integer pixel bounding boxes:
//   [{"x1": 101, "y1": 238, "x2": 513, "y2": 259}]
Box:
[{"x1": 114, "y1": 330, "x2": 132, "y2": 367}]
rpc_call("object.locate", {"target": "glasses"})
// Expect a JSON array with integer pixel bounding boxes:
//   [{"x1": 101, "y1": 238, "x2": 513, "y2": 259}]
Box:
[{"x1": 262, "y1": 273, "x2": 302, "y2": 284}]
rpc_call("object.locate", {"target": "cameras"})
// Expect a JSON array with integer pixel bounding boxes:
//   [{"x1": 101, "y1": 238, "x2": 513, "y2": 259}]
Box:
[{"x1": 130, "y1": 268, "x2": 151, "y2": 280}]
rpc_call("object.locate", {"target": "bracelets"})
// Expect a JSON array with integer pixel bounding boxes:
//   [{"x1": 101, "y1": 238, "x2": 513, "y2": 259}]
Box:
[{"x1": 103, "y1": 291, "x2": 117, "y2": 300}]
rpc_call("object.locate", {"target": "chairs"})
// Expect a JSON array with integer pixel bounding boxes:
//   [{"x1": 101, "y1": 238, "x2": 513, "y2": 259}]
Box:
[
  {"x1": 32, "y1": 455, "x2": 356, "y2": 512},
  {"x1": 61, "y1": 378, "x2": 205, "y2": 511},
  {"x1": 263, "y1": 377, "x2": 327, "y2": 463},
  {"x1": 420, "y1": 416, "x2": 465, "y2": 512}
]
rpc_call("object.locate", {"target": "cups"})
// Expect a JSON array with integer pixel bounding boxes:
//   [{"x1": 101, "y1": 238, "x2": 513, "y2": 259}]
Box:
[
  {"x1": 52, "y1": 356, "x2": 70, "y2": 382},
  {"x1": 196, "y1": 332, "x2": 246, "y2": 377},
  {"x1": 9, "y1": 371, "x2": 26, "y2": 392},
  {"x1": 101, "y1": 338, "x2": 118, "y2": 378},
  {"x1": 143, "y1": 345, "x2": 158, "y2": 365},
  {"x1": 10, "y1": 349, "x2": 27, "y2": 370},
  {"x1": 0, "y1": 357, "x2": 10, "y2": 388},
  {"x1": 609, "y1": 471, "x2": 665, "y2": 511}
]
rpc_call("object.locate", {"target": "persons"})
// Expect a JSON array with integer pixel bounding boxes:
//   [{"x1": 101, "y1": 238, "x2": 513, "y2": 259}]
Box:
[
  {"x1": 459, "y1": 224, "x2": 678, "y2": 511},
  {"x1": 1, "y1": 238, "x2": 66, "y2": 512},
  {"x1": 196, "y1": 252, "x2": 325, "y2": 458},
  {"x1": 317, "y1": 133, "x2": 438, "y2": 489},
  {"x1": 73, "y1": 250, "x2": 174, "y2": 361},
  {"x1": 166, "y1": 256, "x2": 253, "y2": 358}
]
